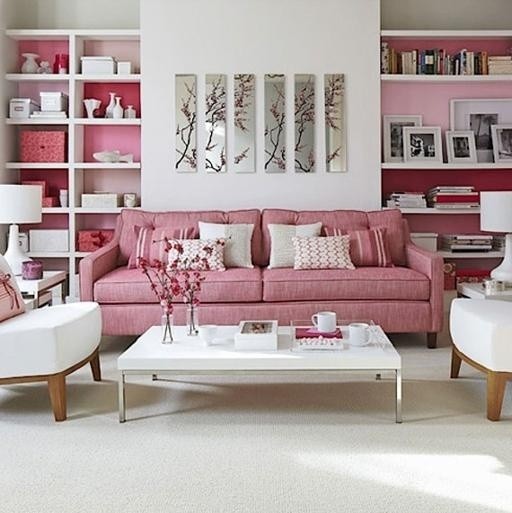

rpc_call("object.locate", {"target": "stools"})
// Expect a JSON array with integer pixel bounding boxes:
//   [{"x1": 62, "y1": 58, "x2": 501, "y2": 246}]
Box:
[{"x1": 449, "y1": 296, "x2": 512, "y2": 420}]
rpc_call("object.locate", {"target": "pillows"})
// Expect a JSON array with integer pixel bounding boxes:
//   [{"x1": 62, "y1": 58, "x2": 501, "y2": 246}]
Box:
[
  {"x1": 290, "y1": 233, "x2": 356, "y2": 271},
  {"x1": 0, "y1": 268, "x2": 24, "y2": 325},
  {"x1": 320, "y1": 221, "x2": 395, "y2": 269},
  {"x1": 196, "y1": 219, "x2": 256, "y2": 268},
  {"x1": 165, "y1": 237, "x2": 226, "y2": 272},
  {"x1": 126, "y1": 222, "x2": 198, "y2": 268},
  {"x1": 264, "y1": 221, "x2": 323, "y2": 268}
]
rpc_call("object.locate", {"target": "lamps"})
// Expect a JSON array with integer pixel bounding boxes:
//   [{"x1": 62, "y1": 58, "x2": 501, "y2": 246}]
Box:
[
  {"x1": 478, "y1": 188, "x2": 512, "y2": 284},
  {"x1": 0, "y1": 183, "x2": 44, "y2": 277}
]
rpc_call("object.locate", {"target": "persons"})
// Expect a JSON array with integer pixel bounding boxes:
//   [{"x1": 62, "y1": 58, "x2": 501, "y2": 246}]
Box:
[{"x1": 248, "y1": 324, "x2": 264, "y2": 332}]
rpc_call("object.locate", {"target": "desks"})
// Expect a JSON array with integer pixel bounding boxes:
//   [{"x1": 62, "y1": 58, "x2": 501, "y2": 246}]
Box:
[
  {"x1": 456, "y1": 281, "x2": 512, "y2": 301},
  {"x1": 12, "y1": 269, "x2": 67, "y2": 309}
]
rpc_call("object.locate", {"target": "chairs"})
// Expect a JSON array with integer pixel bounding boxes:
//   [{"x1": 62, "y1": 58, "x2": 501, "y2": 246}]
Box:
[{"x1": 0, "y1": 253, "x2": 103, "y2": 422}]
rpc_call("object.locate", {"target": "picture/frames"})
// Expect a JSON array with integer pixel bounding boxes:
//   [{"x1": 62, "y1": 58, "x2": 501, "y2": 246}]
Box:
[
  {"x1": 448, "y1": 98, "x2": 512, "y2": 162},
  {"x1": 445, "y1": 129, "x2": 480, "y2": 164},
  {"x1": 401, "y1": 124, "x2": 445, "y2": 164},
  {"x1": 382, "y1": 114, "x2": 422, "y2": 163},
  {"x1": 489, "y1": 122, "x2": 512, "y2": 162}
]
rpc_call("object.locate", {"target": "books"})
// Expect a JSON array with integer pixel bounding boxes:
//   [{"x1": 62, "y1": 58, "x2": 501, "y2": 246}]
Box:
[
  {"x1": 380, "y1": 41, "x2": 512, "y2": 75},
  {"x1": 30, "y1": 111, "x2": 67, "y2": 120},
  {"x1": 388, "y1": 184, "x2": 505, "y2": 253},
  {"x1": 295, "y1": 326, "x2": 344, "y2": 340}
]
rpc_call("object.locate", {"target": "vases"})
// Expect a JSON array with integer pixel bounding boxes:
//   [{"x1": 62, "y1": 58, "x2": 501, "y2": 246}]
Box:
[
  {"x1": 112, "y1": 96, "x2": 124, "y2": 118},
  {"x1": 158, "y1": 299, "x2": 177, "y2": 344},
  {"x1": 124, "y1": 103, "x2": 136, "y2": 118},
  {"x1": 19, "y1": 52, "x2": 40, "y2": 73},
  {"x1": 105, "y1": 90, "x2": 116, "y2": 117}
]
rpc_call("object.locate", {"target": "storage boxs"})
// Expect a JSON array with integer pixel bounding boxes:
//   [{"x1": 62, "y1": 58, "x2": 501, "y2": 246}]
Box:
[
  {"x1": 19, "y1": 130, "x2": 67, "y2": 161},
  {"x1": 78, "y1": 228, "x2": 115, "y2": 251},
  {"x1": 409, "y1": 231, "x2": 439, "y2": 251},
  {"x1": 28, "y1": 228, "x2": 67, "y2": 253},
  {"x1": 38, "y1": 91, "x2": 68, "y2": 111},
  {"x1": 8, "y1": 97, "x2": 38, "y2": 117},
  {"x1": 79, "y1": 55, "x2": 116, "y2": 74}
]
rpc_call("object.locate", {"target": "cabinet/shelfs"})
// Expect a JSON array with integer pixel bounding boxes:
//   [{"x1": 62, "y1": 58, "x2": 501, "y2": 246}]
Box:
[
  {"x1": 381, "y1": 29, "x2": 512, "y2": 260},
  {"x1": 3, "y1": 28, "x2": 141, "y2": 295}
]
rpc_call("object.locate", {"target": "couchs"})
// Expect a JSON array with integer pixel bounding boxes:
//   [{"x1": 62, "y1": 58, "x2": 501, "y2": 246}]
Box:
[{"x1": 77, "y1": 207, "x2": 445, "y2": 348}]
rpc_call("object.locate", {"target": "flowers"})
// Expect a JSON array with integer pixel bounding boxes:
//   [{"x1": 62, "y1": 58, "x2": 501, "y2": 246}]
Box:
[{"x1": 136, "y1": 235, "x2": 214, "y2": 341}]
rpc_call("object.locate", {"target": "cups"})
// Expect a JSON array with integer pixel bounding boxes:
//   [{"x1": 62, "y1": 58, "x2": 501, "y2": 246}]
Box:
[
  {"x1": 348, "y1": 321, "x2": 372, "y2": 347},
  {"x1": 311, "y1": 309, "x2": 336, "y2": 333}
]
